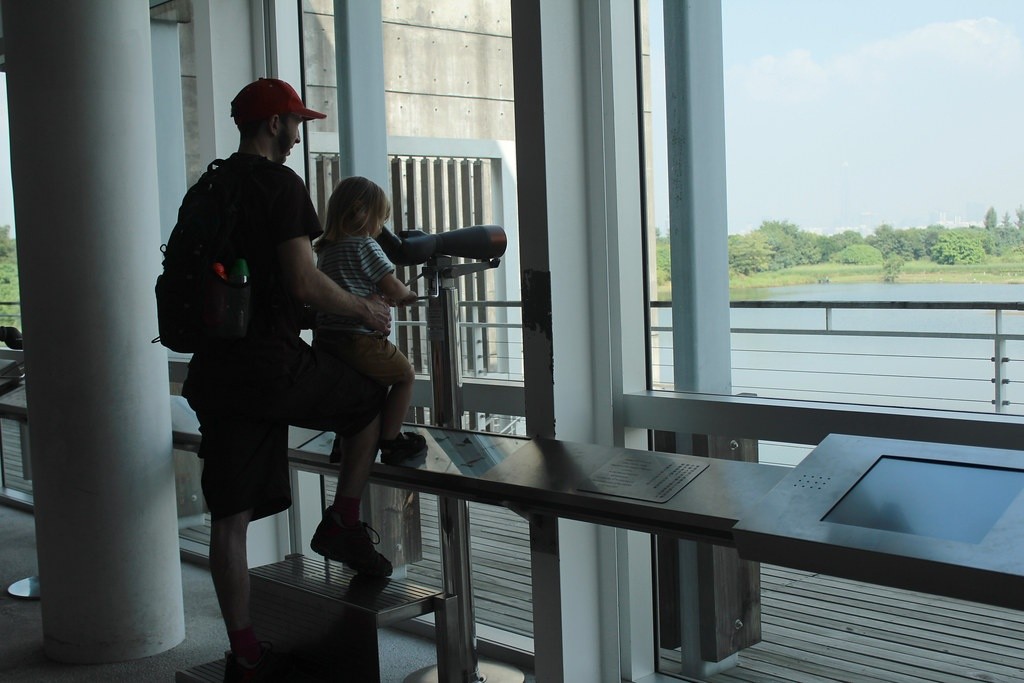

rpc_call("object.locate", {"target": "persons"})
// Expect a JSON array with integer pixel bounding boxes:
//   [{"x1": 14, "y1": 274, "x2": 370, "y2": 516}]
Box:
[
  {"x1": 178, "y1": 78, "x2": 392, "y2": 683},
  {"x1": 312, "y1": 177, "x2": 426, "y2": 465}
]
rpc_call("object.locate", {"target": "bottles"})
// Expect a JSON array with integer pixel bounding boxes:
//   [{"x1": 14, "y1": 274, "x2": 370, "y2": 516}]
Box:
[{"x1": 202, "y1": 262, "x2": 229, "y2": 326}]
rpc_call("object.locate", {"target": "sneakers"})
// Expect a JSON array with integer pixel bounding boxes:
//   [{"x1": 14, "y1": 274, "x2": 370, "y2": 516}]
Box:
[
  {"x1": 223, "y1": 640, "x2": 319, "y2": 683},
  {"x1": 310, "y1": 511, "x2": 393, "y2": 578}
]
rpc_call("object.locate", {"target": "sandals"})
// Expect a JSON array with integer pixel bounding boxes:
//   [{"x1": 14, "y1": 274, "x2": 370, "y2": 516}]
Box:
[
  {"x1": 329, "y1": 437, "x2": 342, "y2": 466},
  {"x1": 378, "y1": 431, "x2": 427, "y2": 463}
]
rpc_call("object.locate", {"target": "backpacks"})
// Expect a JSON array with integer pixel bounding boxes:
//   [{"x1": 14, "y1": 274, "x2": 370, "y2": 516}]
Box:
[{"x1": 156, "y1": 158, "x2": 274, "y2": 353}]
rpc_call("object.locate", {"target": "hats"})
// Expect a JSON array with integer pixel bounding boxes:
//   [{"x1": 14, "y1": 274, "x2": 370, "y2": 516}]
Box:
[{"x1": 231, "y1": 77, "x2": 326, "y2": 124}]
